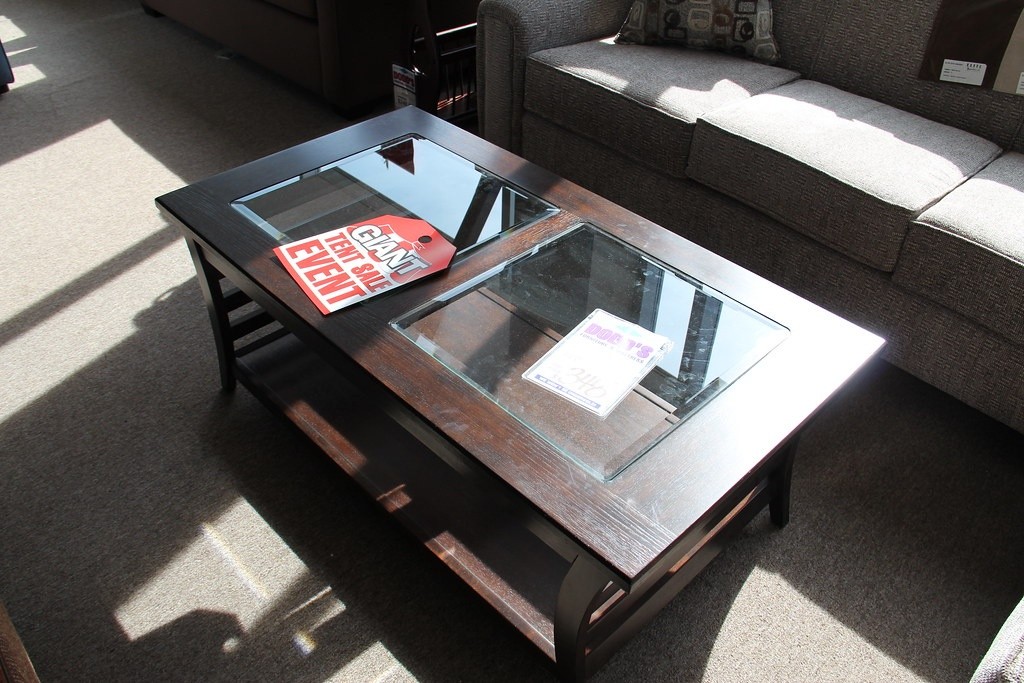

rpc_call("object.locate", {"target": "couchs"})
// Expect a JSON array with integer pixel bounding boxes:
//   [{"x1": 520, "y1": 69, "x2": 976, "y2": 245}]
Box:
[
  {"x1": 476, "y1": 0, "x2": 1024, "y2": 440},
  {"x1": 140, "y1": 0, "x2": 412, "y2": 119}
]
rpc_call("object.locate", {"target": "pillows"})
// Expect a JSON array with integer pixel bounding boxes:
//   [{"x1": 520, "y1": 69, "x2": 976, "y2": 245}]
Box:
[{"x1": 614, "y1": 0, "x2": 781, "y2": 65}]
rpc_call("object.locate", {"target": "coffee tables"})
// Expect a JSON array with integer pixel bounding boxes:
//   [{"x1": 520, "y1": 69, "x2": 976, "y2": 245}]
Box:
[{"x1": 155, "y1": 104, "x2": 891, "y2": 683}]
